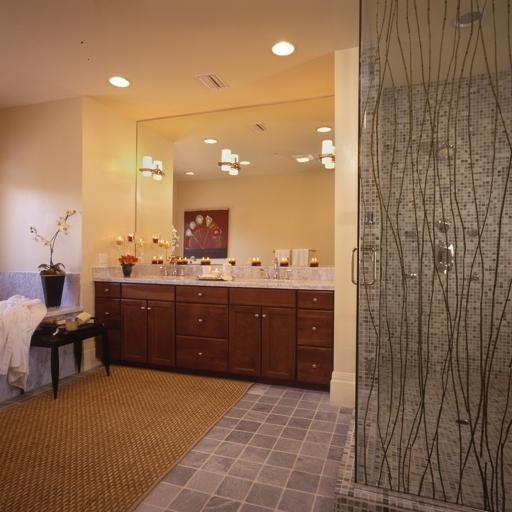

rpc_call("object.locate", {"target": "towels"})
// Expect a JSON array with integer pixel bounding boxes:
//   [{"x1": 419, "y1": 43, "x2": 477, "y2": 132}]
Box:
[
  {"x1": 292, "y1": 248, "x2": 309, "y2": 266},
  {"x1": 275, "y1": 249, "x2": 291, "y2": 267}
]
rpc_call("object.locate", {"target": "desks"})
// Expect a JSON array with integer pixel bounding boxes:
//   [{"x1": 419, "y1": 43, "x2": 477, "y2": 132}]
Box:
[{"x1": 17, "y1": 321, "x2": 113, "y2": 399}]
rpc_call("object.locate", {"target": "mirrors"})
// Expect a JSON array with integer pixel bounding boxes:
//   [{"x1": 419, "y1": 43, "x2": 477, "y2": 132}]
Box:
[{"x1": 134, "y1": 95, "x2": 336, "y2": 269}]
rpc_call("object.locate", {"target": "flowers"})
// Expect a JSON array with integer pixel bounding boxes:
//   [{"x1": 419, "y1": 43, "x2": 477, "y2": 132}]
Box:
[
  {"x1": 151, "y1": 233, "x2": 170, "y2": 261},
  {"x1": 28, "y1": 206, "x2": 79, "y2": 275},
  {"x1": 115, "y1": 231, "x2": 145, "y2": 266},
  {"x1": 185, "y1": 213, "x2": 222, "y2": 249}
]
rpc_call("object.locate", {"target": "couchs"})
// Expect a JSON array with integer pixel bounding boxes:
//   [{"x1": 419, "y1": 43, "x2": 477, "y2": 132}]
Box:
[{"x1": 0, "y1": 270, "x2": 80, "y2": 404}]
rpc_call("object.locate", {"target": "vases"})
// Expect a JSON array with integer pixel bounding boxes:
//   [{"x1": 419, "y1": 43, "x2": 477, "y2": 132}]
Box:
[
  {"x1": 40, "y1": 269, "x2": 67, "y2": 309},
  {"x1": 120, "y1": 263, "x2": 136, "y2": 279}
]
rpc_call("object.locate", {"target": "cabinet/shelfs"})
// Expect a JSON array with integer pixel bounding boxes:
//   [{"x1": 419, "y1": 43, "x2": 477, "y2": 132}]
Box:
[
  {"x1": 228, "y1": 287, "x2": 296, "y2": 383},
  {"x1": 176, "y1": 286, "x2": 229, "y2": 377},
  {"x1": 296, "y1": 290, "x2": 334, "y2": 384},
  {"x1": 94, "y1": 281, "x2": 121, "y2": 362},
  {"x1": 121, "y1": 283, "x2": 176, "y2": 366}
]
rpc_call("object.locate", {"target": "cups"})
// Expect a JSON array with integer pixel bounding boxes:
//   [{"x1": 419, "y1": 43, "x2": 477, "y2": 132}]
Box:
[{"x1": 65, "y1": 321, "x2": 78, "y2": 331}]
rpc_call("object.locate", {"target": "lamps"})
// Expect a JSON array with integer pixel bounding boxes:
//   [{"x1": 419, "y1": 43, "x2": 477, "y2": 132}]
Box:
[
  {"x1": 325, "y1": 146, "x2": 335, "y2": 170},
  {"x1": 319, "y1": 139, "x2": 333, "y2": 165},
  {"x1": 228, "y1": 154, "x2": 241, "y2": 176},
  {"x1": 139, "y1": 155, "x2": 153, "y2": 177},
  {"x1": 152, "y1": 159, "x2": 165, "y2": 181},
  {"x1": 218, "y1": 149, "x2": 230, "y2": 171}
]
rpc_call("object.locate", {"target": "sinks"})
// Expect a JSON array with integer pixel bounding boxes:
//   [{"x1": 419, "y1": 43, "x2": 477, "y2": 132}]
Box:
[{"x1": 256, "y1": 278, "x2": 294, "y2": 284}]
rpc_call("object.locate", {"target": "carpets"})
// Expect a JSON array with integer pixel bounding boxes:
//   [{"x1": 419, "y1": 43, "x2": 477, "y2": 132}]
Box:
[{"x1": 1, "y1": 365, "x2": 254, "y2": 511}]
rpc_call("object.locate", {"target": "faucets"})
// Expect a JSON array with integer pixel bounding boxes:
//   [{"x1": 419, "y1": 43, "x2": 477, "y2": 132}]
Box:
[{"x1": 273, "y1": 258, "x2": 280, "y2": 278}]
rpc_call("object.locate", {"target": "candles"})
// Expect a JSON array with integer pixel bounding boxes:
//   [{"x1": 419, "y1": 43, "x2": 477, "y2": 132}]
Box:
[{"x1": 151, "y1": 255, "x2": 320, "y2": 267}]
